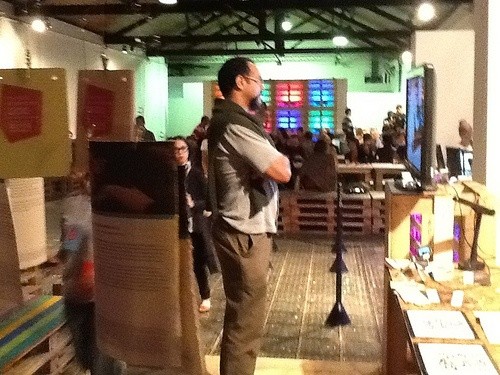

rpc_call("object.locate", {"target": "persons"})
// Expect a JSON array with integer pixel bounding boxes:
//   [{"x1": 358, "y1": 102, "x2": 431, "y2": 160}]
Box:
[
  {"x1": 207, "y1": 57, "x2": 292, "y2": 375},
  {"x1": 132, "y1": 105, "x2": 473, "y2": 313}
]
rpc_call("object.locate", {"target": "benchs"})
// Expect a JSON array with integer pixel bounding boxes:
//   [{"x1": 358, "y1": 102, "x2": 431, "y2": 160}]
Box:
[{"x1": 275, "y1": 190, "x2": 386, "y2": 234}]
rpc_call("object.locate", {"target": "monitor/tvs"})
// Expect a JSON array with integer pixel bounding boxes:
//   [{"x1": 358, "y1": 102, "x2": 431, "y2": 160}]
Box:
[{"x1": 393, "y1": 63, "x2": 438, "y2": 192}]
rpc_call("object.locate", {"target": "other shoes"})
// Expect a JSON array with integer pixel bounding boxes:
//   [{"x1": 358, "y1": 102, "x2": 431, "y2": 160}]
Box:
[{"x1": 199, "y1": 300, "x2": 211, "y2": 312}]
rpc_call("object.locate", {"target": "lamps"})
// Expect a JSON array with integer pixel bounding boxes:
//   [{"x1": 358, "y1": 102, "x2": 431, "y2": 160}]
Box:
[{"x1": 452, "y1": 197, "x2": 495, "y2": 271}]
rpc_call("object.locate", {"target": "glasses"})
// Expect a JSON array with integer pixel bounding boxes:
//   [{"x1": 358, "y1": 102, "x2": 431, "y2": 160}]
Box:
[
  {"x1": 241, "y1": 75, "x2": 264, "y2": 90},
  {"x1": 172, "y1": 146, "x2": 189, "y2": 154}
]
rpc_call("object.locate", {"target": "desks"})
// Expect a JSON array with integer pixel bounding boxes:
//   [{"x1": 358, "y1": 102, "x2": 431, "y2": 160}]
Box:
[
  {"x1": 388, "y1": 259, "x2": 500, "y2": 375},
  {"x1": 293, "y1": 161, "x2": 406, "y2": 190}
]
